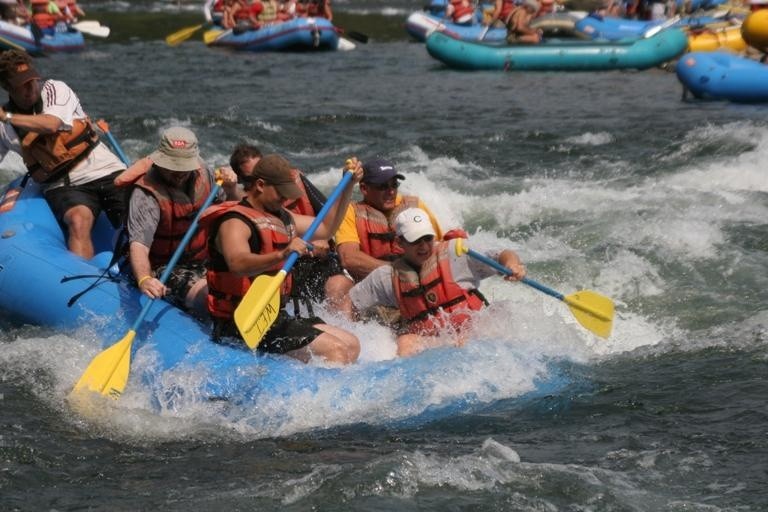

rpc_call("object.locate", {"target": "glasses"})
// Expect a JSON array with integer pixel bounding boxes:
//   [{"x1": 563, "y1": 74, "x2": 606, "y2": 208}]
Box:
[
  {"x1": 400, "y1": 235, "x2": 434, "y2": 244},
  {"x1": 241, "y1": 174, "x2": 255, "y2": 182},
  {"x1": 377, "y1": 183, "x2": 400, "y2": 190}
]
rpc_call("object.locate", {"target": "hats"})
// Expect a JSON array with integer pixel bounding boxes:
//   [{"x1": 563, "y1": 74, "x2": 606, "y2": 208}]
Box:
[
  {"x1": 360, "y1": 158, "x2": 405, "y2": 187},
  {"x1": 1, "y1": 50, "x2": 40, "y2": 89},
  {"x1": 150, "y1": 126, "x2": 202, "y2": 170},
  {"x1": 255, "y1": 153, "x2": 303, "y2": 198},
  {"x1": 395, "y1": 207, "x2": 438, "y2": 243}
]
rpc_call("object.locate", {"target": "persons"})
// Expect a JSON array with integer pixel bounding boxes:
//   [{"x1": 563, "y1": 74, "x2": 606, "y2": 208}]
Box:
[
  {"x1": 201, "y1": 0, "x2": 226, "y2": 28},
  {"x1": 505, "y1": 0, "x2": 544, "y2": 45},
  {"x1": 599, "y1": 0, "x2": 725, "y2": 20},
  {"x1": 230, "y1": 144, "x2": 329, "y2": 219},
  {"x1": 0, "y1": 50, "x2": 134, "y2": 261},
  {"x1": 537, "y1": 0, "x2": 566, "y2": 16},
  {"x1": 286, "y1": 0, "x2": 335, "y2": 21},
  {"x1": 327, "y1": 158, "x2": 444, "y2": 317},
  {"x1": 0, "y1": 0, "x2": 85, "y2": 37},
  {"x1": 116, "y1": 126, "x2": 228, "y2": 313},
  {"x1": 444, "y1": 0, "x2": 475, "y2": 26},
  {"x1": 229, "y1": 0, "x2": 253, "y2": 29},
  {"x1": 249, "y1": 0, "x2": 289, "y2": 31},
  {"x1": 206, "y1": 153, "x2": 361, "y2": 366},
  {"x1": 339, "y1": 207, "x2": 527, "y2": 357},
  {"x1": 223, "y1": 0, "x2": 233, "y2": 29},
  {"x1": 482, "y1": 0, "x2": 513, "y2": 28}
]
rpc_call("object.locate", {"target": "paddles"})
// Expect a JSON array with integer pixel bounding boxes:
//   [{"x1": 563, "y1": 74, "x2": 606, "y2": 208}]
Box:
[
  {"x1": 478, "y1": 25, "x2": 489, "y2": 42},
  {"x1": 424, "y1": 16, "x2": 447, "y2": 37},
  {"x1": 165, "y1": 16, "x2": 222, "y2": 47},
  {"x1": 71, "y1": 169, "x2": 228, "y2": 400},
  {"x1": 69, "y1": 21, "x2": 101, "y2": 27},
  {"x1": 68, "y1": 26, "x2": 111, "y2": 37},
  {"x1": 233, "y1": 158, "x2": 360, "y2": 350},
  {"x1": 203, "y1": 28, "x2": 233, "y2": 44},
  {"x1": 455, "y1": 236, "x2": 615, "y2": 339},
  {"x1": 334, "y1": 26, "x2": 369, "y2": 44}
]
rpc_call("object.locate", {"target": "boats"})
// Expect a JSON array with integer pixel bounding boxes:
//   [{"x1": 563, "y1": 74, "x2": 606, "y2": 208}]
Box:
[
  {"x1": 425, "y1": 30, "x2": 689, "y2": 70},
  {"x1": 573, "y1": 0, "x2": 725, "y2": 39},
  {"x1": 0, "y1": 20, "x2": 84, "y2": 54},
  {"x1": 686, "y1": 20, "x2": 744, "y2": 52},
  {"x1": 407, "y1": 1, "x2": 511, "y2": 40},
  {"x1": 675, "y1": 54, "x2": 768, "y2": 96},
  {"x1": 1, "y1": 181, "x2": 566, "y2": 431},
  {"x1": 209, "y1": 17, "x2": 338, "y2": 50}
]
